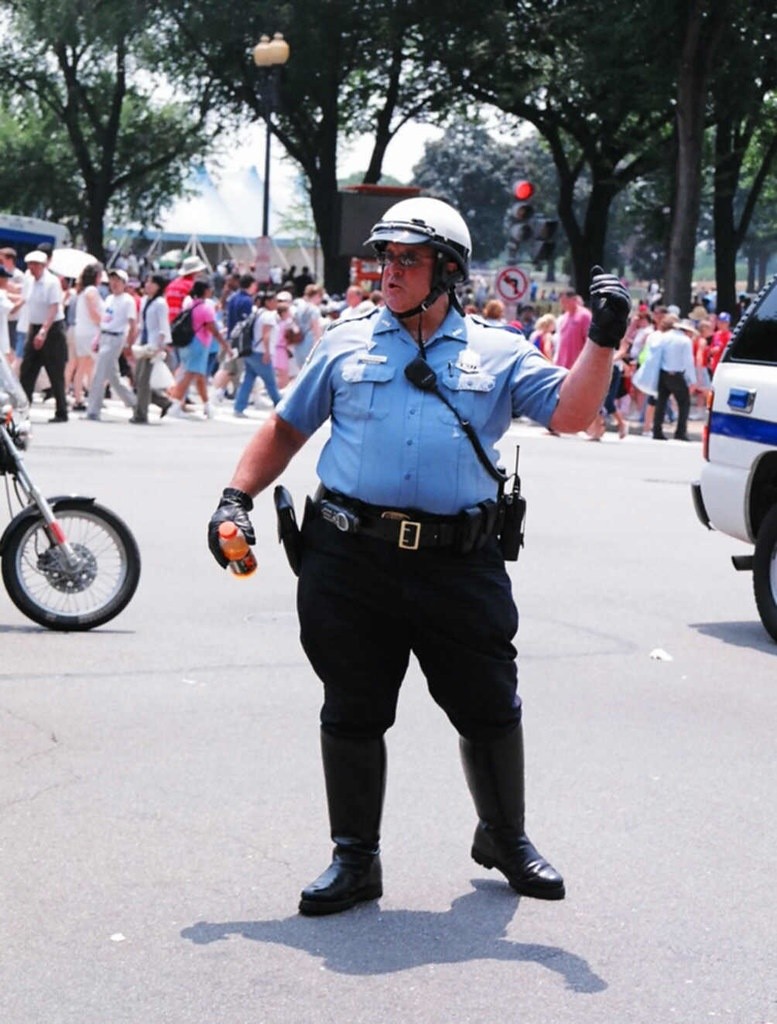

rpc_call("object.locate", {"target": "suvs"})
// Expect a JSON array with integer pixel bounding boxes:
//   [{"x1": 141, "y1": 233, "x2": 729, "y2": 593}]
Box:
[{"x1": 689, "y1": 273, "x2": 777, "y2": 641}]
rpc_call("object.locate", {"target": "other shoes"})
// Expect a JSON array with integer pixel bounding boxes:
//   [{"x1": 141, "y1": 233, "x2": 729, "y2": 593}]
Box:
[
  {"x1": 544, "y1": 403, "x2": 707, "y2": 441},
  {"x1": 18, "y1": 380, "x2": 293, "y2": 425}
]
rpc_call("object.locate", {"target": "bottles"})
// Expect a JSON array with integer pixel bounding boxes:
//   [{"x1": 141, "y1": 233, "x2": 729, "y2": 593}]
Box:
[{"x1": 217, "y1": 520, "x2": 258, "y2": 578}]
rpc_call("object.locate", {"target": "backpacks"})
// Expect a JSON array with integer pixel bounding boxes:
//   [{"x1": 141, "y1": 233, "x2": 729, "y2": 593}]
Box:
[
  {"x1": 170, "y1": 302, "x2": 200, "y2": 347},
  {"x1": 238, "y1": 312, "x2": 267, "y2": 356}
]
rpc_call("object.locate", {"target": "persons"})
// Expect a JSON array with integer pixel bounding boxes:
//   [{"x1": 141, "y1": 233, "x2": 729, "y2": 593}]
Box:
[
  {"x1": 206, "y1": 198, "x2": 634, "y2": 916},
  {"x1": 1, "y1": 241, "x2": 233, "y2": 423},
  {"x1": 612, "y1": 291, "x2": 751, "y2": 442},
  {"x1": 454, "y1": 274, "x2": 631, "y2": 441},
  {"x1": 211, "y1": 258, "x2": 384, "y2": 419}
]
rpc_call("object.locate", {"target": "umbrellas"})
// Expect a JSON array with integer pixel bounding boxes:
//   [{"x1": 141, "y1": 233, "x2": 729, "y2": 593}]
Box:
[{"x1": 49, "y1": 247, "x2": 109, "y2": 286}]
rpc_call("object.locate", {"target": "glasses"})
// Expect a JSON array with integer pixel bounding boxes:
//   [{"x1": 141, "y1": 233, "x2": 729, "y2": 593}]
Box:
[{"x1": 375, "y1": 251, "x2": 436, "y2": 268}]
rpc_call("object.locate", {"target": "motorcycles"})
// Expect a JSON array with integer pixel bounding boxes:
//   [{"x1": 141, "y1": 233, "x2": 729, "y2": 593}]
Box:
[{"x1": 0, "y1": 348, "x2": 143, "y2": 632}]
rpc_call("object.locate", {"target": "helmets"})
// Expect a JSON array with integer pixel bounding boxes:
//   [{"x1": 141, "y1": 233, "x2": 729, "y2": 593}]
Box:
[{"x1": 362, "y1": 196, "x2": 472, "y2": 276}]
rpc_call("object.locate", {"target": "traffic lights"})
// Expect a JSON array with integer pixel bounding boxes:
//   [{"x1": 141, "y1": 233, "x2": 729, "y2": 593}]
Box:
[
  {"x1": 530, "y1": 213, "x2": 560, "y2": 264},
  {"x1": 503, "y1": 178, "x2": 535, "y2": 247}
]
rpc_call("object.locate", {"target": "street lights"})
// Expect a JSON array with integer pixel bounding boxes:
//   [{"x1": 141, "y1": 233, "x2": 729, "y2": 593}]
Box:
[{"x1": 253, "y1": 32, "x2": 291, "y2": 290}]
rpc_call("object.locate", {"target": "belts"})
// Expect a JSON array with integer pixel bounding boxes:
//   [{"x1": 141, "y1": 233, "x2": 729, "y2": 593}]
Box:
[
  {"x1": 660, "y1": 369, "x2": 683, "y2": 375},
  {"x1": 101, "y1": 330, "x2": 123, "y2": 335},
  {"x1": 324, "y1": 490, "x2": 461, "y2": 550}
]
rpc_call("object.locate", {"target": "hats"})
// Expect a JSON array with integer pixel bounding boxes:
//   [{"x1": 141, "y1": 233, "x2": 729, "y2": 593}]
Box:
[
  {"x1": 688, "y1": 306, "x2": 708, "y2": 320},
  {"x1": 179, "y1": 255, "x2": 206, "y2": 276},
  {"x1": 0, "y1": 266, "x2": 13, "y2": 277},
  {"x1": 276, "y1": 291, "x2": 292, "y2": 302},
  {"x1": 718, "y1": 312, "x2": 730, "y2": 322},
  {"x1": 667, "y1": 305, "x2": 680, "y2": 316},
  {"x1": 109, "y1": 269, "x2": 128, "y2": 284},
  {"x1": 672, "y1": 318, "x2": 699, "y2": 335},
  {"x1": 25, "y1": 250, "x2": 47, "y2": 264}
]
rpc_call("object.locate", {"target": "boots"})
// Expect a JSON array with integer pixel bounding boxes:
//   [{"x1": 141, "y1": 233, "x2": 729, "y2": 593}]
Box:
[
  {"x1": 459, "y1": 721, "x2": 564, "y2": 900},
  {"x1": 299, "y1": 728, "x2": 387, "y2": 915}
]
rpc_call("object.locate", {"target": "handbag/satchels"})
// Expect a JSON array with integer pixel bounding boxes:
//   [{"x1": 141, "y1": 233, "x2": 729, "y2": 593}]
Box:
[
  {"x1": 284, "y1": 329, "x2": 303, "y2": 344},
  {"x1": 129, "y1": 342, "x2": 155, "y2": 360}
]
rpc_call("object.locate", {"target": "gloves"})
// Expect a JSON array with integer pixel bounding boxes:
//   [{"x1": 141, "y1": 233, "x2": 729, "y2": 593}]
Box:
[
  {"x1": 587, "y1": 265, "x2": 630, "y2": 350},
  {"x1": 208, "y1": 489, "x2": 256, "y2": 569}
]
